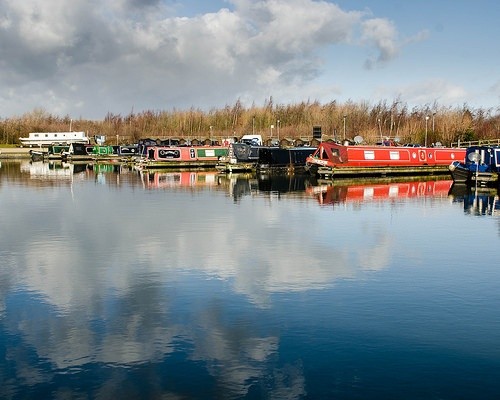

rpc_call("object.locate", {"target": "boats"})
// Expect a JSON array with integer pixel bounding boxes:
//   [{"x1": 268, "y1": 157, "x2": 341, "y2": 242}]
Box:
[
  {"x1": 305, "y1": 141, "x2": 468, "y2": 177},
  {"x1": 29, "y1": 134, "x2": 318, "y2": 173},
  {"x1": 19, "y1": 131, "x2": 90, "y2": 148},
  {"x1": 447, "y1": 145, "x2": 500, "y2": 194}
]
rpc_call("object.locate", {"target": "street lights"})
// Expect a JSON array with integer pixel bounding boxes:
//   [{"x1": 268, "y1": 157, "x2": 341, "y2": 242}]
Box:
[
  {"x1": 425, "y1": 115, "x2": 430, "y2": 147},
  {"x1": 343, "y1": 115, "x2": 347, "y2": 140},
  {"x1": 432, "y1": 112, "x2": 436, "y2": 143}
]
